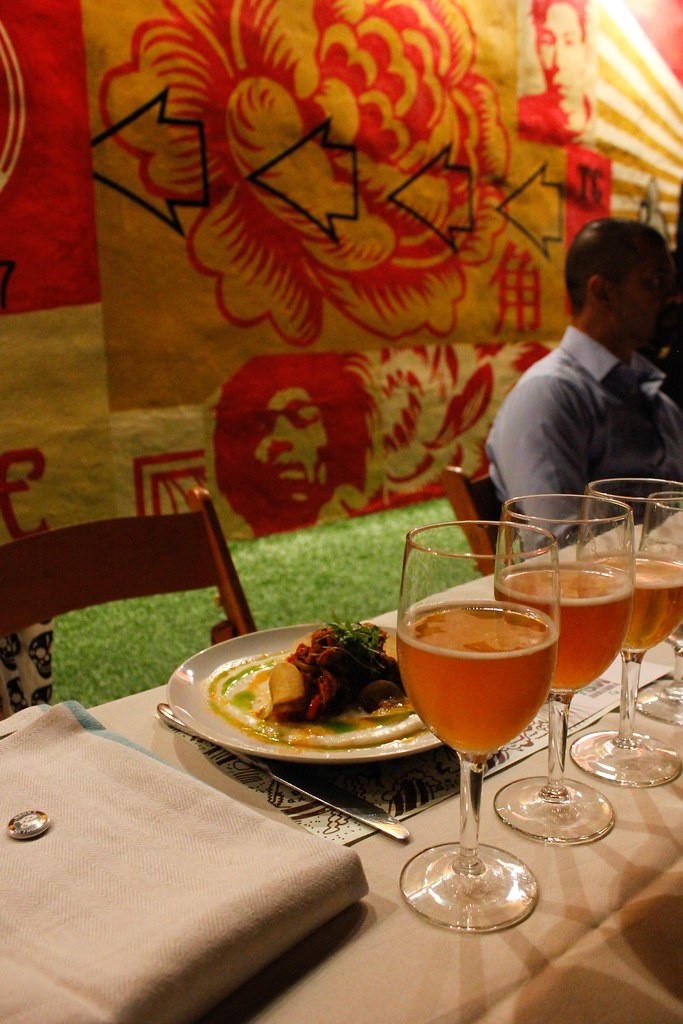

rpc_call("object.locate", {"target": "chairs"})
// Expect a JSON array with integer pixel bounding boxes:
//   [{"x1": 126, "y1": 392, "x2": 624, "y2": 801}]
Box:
[
  {"x1": 441, "y1": 465, "x2": 502, "y2": 578},
  {"x1": 0, "y1": 485, "x2": 258, "y2": 723}
]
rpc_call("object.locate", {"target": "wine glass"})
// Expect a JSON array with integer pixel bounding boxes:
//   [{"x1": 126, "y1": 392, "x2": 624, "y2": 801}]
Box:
[
  {"x1": 394, "y1": 519, "x2": 560, "y2": 935},
  {"x1": 493, "y1": 492, "x2": 636, "y2": 848},
  {"x1": 634, "y1": 491, "x2": 683, "y2": 730},
  {"x1": 568, "y1": 479, "x2": 683, "y2": 791}
]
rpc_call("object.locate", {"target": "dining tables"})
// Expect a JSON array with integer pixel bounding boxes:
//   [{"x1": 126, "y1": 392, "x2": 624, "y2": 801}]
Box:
[{"x1": 90, "y1": 525, "x2": 683, "y2": 1024}]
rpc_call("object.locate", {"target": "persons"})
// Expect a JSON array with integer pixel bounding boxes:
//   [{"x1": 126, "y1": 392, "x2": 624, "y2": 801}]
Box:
[{"x1": 483, "y1": 189, "x2": 683, "y2": 561}]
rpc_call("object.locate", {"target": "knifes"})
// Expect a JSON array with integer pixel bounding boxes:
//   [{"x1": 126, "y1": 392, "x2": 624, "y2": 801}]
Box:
[{"x1": 156, "y1": 703, "x2": 410, "y2": 842}]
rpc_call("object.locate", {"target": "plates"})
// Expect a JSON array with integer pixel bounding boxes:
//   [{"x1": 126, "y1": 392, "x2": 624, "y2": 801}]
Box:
[{"x1": 167, "y1": 622, "x2": 444, "y2": 766}]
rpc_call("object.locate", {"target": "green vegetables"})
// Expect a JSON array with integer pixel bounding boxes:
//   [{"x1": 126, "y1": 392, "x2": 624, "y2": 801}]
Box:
[{"x1": 314, "y1": 609, "x2": 386, "y2": 674}]
